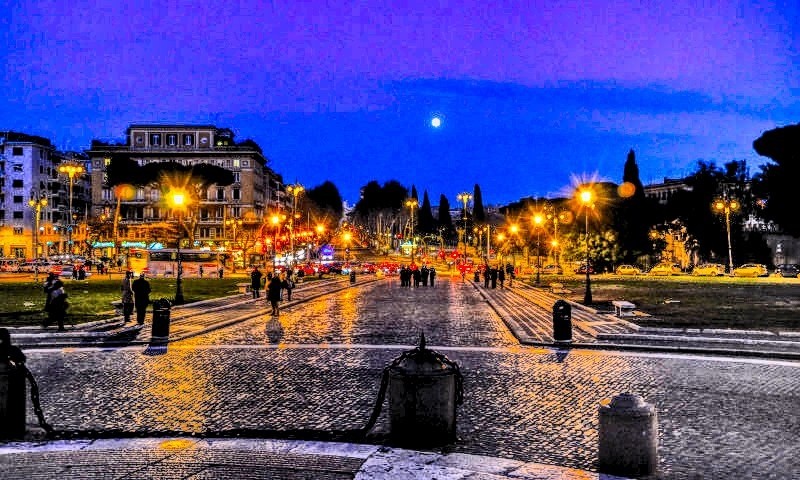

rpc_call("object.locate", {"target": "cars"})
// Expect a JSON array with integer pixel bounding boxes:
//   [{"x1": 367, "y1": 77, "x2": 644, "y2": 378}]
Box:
[
  {"x1": 615, "y1": 265, "x2": 642, "y2": 276},
  {"x1": 690, "y1": 263, "x2": 726, "y2": 277},
  {"x1": 731, "y1": 264, "x2": 768, "y2": 278},
  {"x1": 775, "y1": 263, "x2": 800, "y2": 279},
  {"x1": 245, "y1": 242, "x2": 335, "y2": 268},
  {"x1": 574, "y1": 264, "x2": 594, "y2": 274},
  {"x1": 523, "y1": 263, "x2": 564, "y2": 275},
  {"x1": 649, "y1": 261, "x2": 681, "y2": 277},
  {"x1": 0, "y1": 253, "x2": 87, "y2": 278}
]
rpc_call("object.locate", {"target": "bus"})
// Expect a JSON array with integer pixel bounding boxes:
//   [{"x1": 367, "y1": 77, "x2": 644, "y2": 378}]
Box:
[{"x1": 125, "y1": 247, "x2": 234, "y2": 279}]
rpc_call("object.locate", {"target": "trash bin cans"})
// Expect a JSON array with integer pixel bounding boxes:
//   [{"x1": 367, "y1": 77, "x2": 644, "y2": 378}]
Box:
[
  {"x1": 552, "y1": 298, "x2": 573, "y2": 341},
  {"x1": 349, "y1": 271, "x2": 356, "y2": 282},
  {"x1": 474, "y1": 270, "x2": 480, "y2": 282},
  {"x1": 151, "y1": 298, "x2": 172, "y2": 339}
]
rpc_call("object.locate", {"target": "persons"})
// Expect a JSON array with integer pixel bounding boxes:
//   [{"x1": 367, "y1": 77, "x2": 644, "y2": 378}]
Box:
[
  {"x1": 84, "y1": 256, "x2": 122, "y2": 274},
  {"x1": 78, "y1": 267, "x2": 85, "y2": 280},
  {"x1": 121, "y1": 271, "x2": 133, "y2": 322},
  {"x1": 42, "y1": 272, "x2": 69, "y2": 332},
  {"x1": 264, "y1": 272, "x2": 295, "y2": 317},
  {"x1": 132, "y1": 274, "x2": 151, "y2": 324},
  {"x1": 251, "y1": 267, "x2": 262, "y2": 299},
  {"x1": 483, "y1": 263, "x2": 514, "y2": 289},
  {"x1": 72, "y1": 268, "x2": 77, "y2": 279},
  {"x1": 283, "y1": 269, "x2": 304, "y2": 283},
  {"x1": 400, "y1": 265, "x2": 436, "y2": 288}
]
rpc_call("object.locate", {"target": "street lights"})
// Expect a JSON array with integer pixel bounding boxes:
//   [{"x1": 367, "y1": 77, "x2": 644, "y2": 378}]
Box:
[
  {"x1": 287, "y1": 185, "x2": 304, "y2": 241},
  {"x1": 59, "y1": 165, "x2": 82, "y2": 224},
  {"x1": 533, "y1": 215, "x2": 543, "y2": 285},
  {"x1": 406, "y1": 200, "x2": 418, "y2": 262},
  {"x1": 28, "y1": 198, "x2": 48, "y2": 281},
  {"x1": 578, "y1": 189, "x2": 595, "y2": 306},
  {"x1": 456, "y1": 192, "x2": 472, "y2": 264},
  {"x1": 270, "y1": 215, "x2": 280, "y2": 258},
  {"x1": 713, "y1": 190, "x2": 738, "y2": 277},
  {"x1": 171, "y1": 190, "x2": 188, "y2": 305}
]
